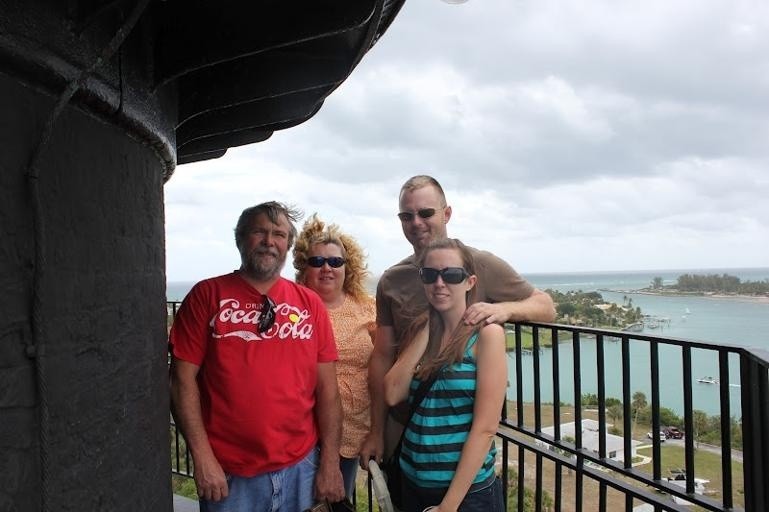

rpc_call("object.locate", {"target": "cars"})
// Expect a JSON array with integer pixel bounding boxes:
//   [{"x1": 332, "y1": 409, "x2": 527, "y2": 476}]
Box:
[{"x1": 647, "y1": 426, "x2": 683, "y2": 442}]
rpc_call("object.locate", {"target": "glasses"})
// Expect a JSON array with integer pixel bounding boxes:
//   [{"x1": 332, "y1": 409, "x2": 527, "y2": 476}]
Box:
[
  {"x1": 259, "y1": 294, "x2": 277, "y2": 333},
  {"x1": 307, "y1": 256, "x2": 347, "y2": 268},
  {"x1": 418, "y1": 266, "x2": 470, "y2": 284},
  {"x1": 397, "y1": 208, "x2": 436, "y2": 222}
]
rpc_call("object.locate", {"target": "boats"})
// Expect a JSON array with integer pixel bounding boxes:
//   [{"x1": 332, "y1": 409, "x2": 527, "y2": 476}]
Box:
[{"x1": 697, "y1": 375, "x2": 719, "y2": 384}]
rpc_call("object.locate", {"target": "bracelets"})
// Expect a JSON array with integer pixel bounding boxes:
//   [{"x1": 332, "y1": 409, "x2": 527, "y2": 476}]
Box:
[{"x1": 422, "y1": 505, "x2": 437, "y2": 512}]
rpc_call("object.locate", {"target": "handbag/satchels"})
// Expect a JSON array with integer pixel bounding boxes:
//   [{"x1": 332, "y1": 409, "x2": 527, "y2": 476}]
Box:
[{"x1": 378, "y1": 399, "x2": 413, "y2": 470}]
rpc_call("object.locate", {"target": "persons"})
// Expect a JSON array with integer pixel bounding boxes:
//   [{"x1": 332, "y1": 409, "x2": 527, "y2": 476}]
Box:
[
  {"x1": 168, "y1": 198, "x2": 347, "y2": 511},
  {"x1": 381, "y1": 236, "x2": 511, "y2": 512},
  {"x1": 356, "y1": 173, "x2": 558, "y2": 512},
  {"x1": 291, "y1": 212, "x2": 379, "y2": 511}
]
